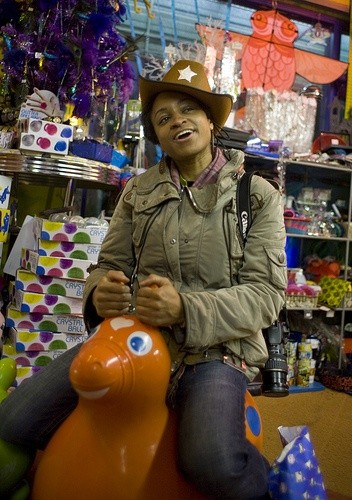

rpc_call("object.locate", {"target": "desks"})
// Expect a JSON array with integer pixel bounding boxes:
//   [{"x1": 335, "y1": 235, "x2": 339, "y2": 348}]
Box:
[{"x1": 0, "y1": 151, "x2": 120, "y2": 220}]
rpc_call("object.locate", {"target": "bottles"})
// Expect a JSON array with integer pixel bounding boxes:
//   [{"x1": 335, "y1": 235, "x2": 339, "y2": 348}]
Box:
[{"x1": 49, "y1": 214, "x2": 109, "y2": 227}]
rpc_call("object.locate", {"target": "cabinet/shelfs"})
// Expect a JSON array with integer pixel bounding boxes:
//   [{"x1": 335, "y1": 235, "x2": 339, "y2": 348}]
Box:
[{"x1": 237, "y1": 144, "x2": 352, "y2": 392}]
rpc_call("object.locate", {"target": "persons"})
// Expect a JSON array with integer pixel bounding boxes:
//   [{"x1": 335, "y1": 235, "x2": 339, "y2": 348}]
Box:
[{"x1": 0, "y1": 60, "x2": 287, "y2": 500}]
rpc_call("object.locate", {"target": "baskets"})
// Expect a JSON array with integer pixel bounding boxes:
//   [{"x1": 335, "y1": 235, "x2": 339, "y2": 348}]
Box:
[{"x1": 284, "y1": 210, "x2": 311, "y2": 234}]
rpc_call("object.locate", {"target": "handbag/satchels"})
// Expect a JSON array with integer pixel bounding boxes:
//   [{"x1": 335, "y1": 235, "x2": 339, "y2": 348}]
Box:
[{"x1": 269, "y1": 425, "x2": 326, "y2": 500}]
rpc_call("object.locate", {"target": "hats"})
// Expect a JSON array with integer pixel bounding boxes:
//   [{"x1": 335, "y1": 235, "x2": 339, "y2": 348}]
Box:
[{"x1": 140, "y1": 60, "x2": 233, "y2": 145}]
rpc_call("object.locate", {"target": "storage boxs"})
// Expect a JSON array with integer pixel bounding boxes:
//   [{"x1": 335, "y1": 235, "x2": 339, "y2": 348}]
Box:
[
  {"x1": 10, "y1": 117, "x2": 128, "y2": 167},
  {"x1": 0, "y1": 219, "x2": 112, "y2": 388}
]
